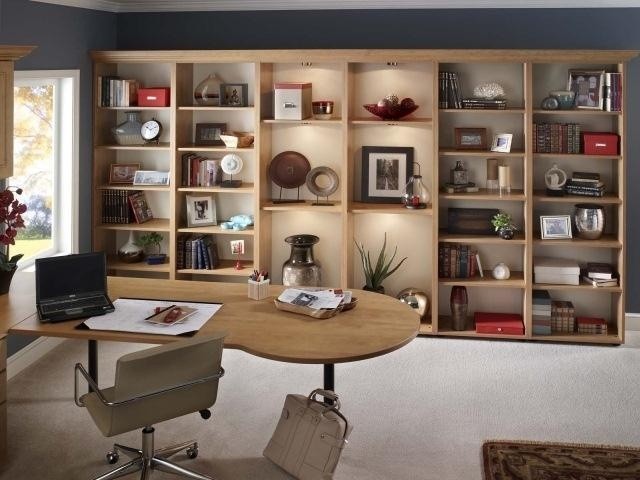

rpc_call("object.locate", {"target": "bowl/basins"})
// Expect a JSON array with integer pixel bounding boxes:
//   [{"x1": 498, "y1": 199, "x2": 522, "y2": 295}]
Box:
[
  {"x1": 363, "y1": 103, "x2": 419, "y2": 120},
  {"x1": 218, "y1": 130, "x2": 254, "y2": 148},
  {"x1": 548, "y1": 90, "x2": 575, "y2": 109}
]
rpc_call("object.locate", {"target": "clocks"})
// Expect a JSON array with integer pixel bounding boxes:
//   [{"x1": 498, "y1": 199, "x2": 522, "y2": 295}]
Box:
[{"x1": 140, "y1": 115, "x2": 163, "y2": 142}]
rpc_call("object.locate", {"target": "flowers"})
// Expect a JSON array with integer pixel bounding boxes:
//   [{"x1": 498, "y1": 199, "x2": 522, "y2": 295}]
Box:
[{"x1": 0, "y1": 183, "x2": 30, "y2": 272}]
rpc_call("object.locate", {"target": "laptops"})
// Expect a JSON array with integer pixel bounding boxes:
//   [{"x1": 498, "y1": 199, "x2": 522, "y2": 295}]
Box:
[{"x1": 36, "y1": 251, "x2": 115, "y2": 323}]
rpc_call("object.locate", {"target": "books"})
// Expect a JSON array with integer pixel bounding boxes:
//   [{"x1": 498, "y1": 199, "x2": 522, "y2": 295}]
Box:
[
  {"x1": 177, "y1": 235, "x2": 220, "y2": 271},
  {"x1": 97, "y1": 76, "x2": 142, "y2": 107},
  {"x1": 532, "y1": 290, "x2": 608, "y2": 336},
  {"x1": 144, "y1": 304, "x2": 199, "y2": 327},
  {"x1": 182, "y1": 154, "x2": 223, "y2": 188},
  {"x1": 439, "y1": 243, "x2": 484, "y2": 280},
  {"x1": 438, "y1": 71, "x2": 507, "y2": 110},
  {"x1": 532, "y1": 122, "x2": 581, "y2": 154},
  {"x1": 533, "y1": 255, "x2": 617, "y2": 288},
  {"x1": 605, "y1": 72, "x2": 622, "y2": 112},
  {"x1": 565, "y1": 170, "x2": 609, "y2": 197},
  {"x1": 102, "y1": 190, "x2": 153, "y2": 225}
]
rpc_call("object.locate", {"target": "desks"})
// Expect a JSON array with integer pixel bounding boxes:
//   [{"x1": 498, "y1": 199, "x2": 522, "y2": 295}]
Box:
[{"x1": 0, "y1": 274, "x2": 424, "y2": 474}]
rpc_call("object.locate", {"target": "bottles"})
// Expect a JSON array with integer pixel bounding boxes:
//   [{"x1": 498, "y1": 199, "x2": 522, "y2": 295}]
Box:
[
  {"x1": 118, "y1": 230, "x2": 144, "y2": 263},
  {"x1": 570, "y1": 203, "x2": 606, "y2": 241},
  {"x1": 402, "y1": 175, "x2": 432, "y2": 210},
  {"x1": 491, "y1": 262, "x2": 512, "y2": 279},
  {"x1": 111, "y1": 112, "x2": 152, "y2": 145},
  {"x1": 450, "y1": 160, "x2": 470, "y2": 185},
  {"x1": 193, "y1": 73, "x2": 226, "y2": 107}
]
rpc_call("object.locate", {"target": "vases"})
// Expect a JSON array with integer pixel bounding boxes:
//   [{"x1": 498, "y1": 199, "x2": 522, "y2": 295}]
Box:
[
  {"x1": 0, "y1": 264, "x2": 19, "y2": 294},
  {"x1": 110, "y1": 108, "x2": 147, "y2": 145},
  {"x1": 117, "y1": 227, "x2": 143, "y2": 264},
  {"x1": 192, "y1": 71, "x2": 230, "y2": 106}
]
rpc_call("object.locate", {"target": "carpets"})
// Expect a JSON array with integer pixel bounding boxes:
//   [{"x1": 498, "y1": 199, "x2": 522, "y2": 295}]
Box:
[{"x1": 481, "y1": 437, "x2": 640, "y2": 478}]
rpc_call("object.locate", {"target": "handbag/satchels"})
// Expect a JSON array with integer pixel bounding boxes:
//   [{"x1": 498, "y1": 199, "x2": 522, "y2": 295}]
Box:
[{"x1": 262, "y1": 387, "x2": 354, "y2": 480}]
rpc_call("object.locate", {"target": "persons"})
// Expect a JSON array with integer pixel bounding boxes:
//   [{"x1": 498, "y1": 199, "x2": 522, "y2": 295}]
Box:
[{"x1": 229, "y1": 89, "x2": 240, "y2": 104}]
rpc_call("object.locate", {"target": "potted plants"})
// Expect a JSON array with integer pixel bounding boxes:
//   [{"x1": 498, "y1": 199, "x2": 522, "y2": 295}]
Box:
[
  {"x1": 491, "y1": 211, "x2": 518, "y2": 240},
  {"x1": 135, "y1": 230, "x2": 168, "y2": 265},
  {"x1": 351, "y1": 230, "x2": 409, "y2": 297}
]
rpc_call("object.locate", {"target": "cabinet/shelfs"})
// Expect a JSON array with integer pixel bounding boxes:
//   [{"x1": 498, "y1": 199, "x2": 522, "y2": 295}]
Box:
[{"x1": 87, "y1": 39, "x2": 639, "y2": 349}]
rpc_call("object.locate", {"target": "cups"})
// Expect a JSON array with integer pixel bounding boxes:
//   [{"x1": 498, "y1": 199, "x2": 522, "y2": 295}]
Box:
[
  {"x1": 220, "y1": 221, "x2": 246, "y2": 232},
  {"x1": 311, "y1": 99, "x2": 334, "y2": 120}
]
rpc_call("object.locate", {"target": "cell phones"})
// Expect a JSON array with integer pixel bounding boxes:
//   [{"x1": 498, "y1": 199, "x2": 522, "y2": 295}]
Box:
[{"x1": 165, "y1": 308, "x2": 180, "y2": 323}]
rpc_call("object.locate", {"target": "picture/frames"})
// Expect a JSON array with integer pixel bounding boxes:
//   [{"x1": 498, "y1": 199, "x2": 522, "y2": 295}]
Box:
[
  {"x1": 361, "y1": 145, "x2": 416, "y2": 205},
  {"x1": 490, "y1": 132, "x2": 514, "y2": 154},
  {"x1": 132, "y1": 169, "x2": 170, "y2": 186},
  {"x1": 538, "y1": 214, "x2": 574, "y2": 240},
  {"x1": 185, "y1": 191, "x2": 218, "y2": 227},
  {"x1": 453, "y1": 127, "x2": 488, "y2": 151},
  {"x1": 565, "y1": 65, "x2": 606, "y2": 113},
  {"x1": 193, "y1": 120, "x2": 226, "y2": 146},
  {"x1": 108, "y1": 161, "x2": 141, "y2": 185}
]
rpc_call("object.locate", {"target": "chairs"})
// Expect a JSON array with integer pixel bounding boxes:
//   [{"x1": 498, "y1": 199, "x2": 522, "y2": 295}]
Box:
[{"x1": 69, "y1": 328, "x2": 229, "y2": 480}]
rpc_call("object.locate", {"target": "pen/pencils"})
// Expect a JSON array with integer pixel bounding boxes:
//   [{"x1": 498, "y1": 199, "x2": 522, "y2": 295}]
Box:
[{"x1": 250, "y1": 270, "x2": 268, "y2": 282}]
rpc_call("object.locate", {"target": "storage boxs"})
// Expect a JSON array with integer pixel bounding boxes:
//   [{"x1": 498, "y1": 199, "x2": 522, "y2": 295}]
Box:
[
  {"x1": 272, "y1": 81, "x2": 314, "y2": 120},
  {"x1": 135, "y1": 87, "x2": 170, "y2": 107},
  {"x1": 533, "y1": 256, "x2": 582, "y2": 286},
  {"x1": 579, "y1": 129, "x2": 620, "y2": 156}
]
